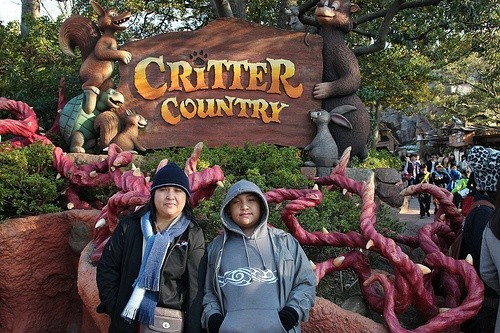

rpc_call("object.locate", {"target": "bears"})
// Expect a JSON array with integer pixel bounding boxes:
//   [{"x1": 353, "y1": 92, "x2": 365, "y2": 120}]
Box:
[{"x1": 298, "y1": 0, "x2": 371, "y2": 161}]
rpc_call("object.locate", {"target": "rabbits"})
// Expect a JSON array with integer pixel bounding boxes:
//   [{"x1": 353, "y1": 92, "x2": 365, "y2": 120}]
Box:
[{"x1": 303, "y1": 105, "x2": 357, "y2": 167}]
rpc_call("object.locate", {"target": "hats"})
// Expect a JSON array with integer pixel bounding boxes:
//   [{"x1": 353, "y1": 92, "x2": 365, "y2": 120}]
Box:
[
  {"x1": 468, "y1": 145, "x2": 500, "y2": 192},
  {"x1": 151, "y1": 163, "x2": 191, "y2": 197}
]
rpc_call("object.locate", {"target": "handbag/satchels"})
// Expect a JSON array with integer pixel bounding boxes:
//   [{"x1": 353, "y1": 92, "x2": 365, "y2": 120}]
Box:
[{"x1": 139, "y1": 306, "x2": 185, "y2": 333}]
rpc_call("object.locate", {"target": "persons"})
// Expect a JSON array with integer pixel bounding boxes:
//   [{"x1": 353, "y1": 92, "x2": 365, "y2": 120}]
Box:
[
  {"x1": 459, "y1": 146, "x2": 500, "y2": 333},
  {"x1": 400, "y1": 152, "x2": 471, "y2": 219},
  {"x1": 201, "y1": 179, "x2": 316, "y2": 333},
  {"x1": 480, "y1": 176, "x2": 500, "y2": 333},
  {"x1": 95, "y1": 163, "x2": 208, "y2": 333}
]
